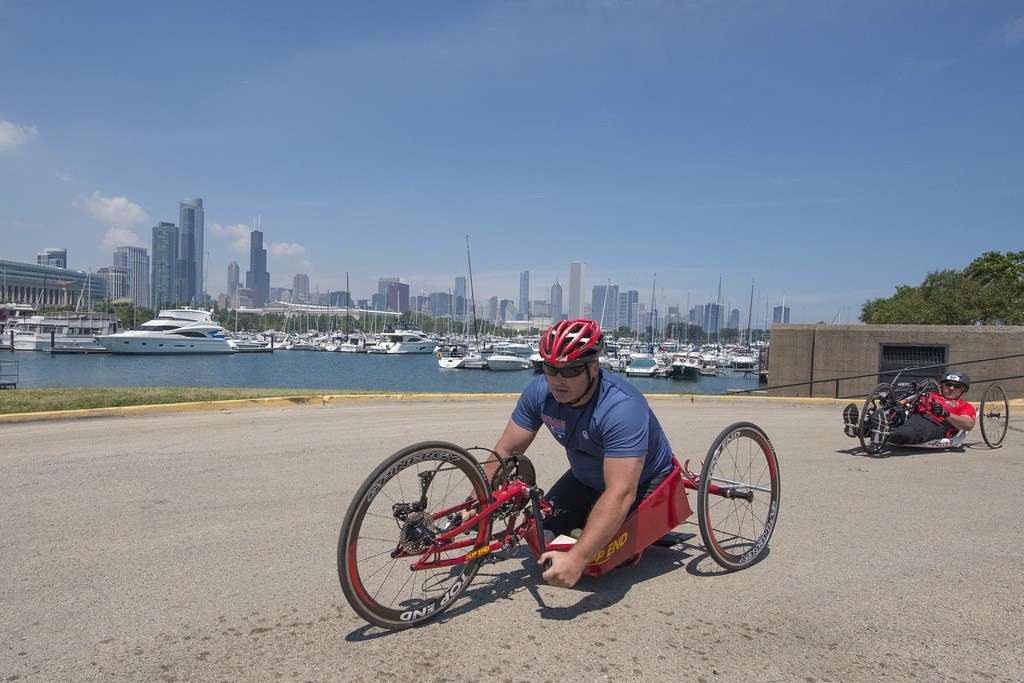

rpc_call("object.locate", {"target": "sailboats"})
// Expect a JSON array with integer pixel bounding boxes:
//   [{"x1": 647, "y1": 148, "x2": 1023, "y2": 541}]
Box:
[
  {"x1": 228, "y1": 235, "x2": 768, "y2": 382},
  {"x1": 0, "y1": 265, "x2": 126, "y2": 359}
]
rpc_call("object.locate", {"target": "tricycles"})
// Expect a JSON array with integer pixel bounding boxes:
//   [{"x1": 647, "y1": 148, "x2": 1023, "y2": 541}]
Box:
[
  {"x1": 842, "y1": 376, "x2": 1010, "y2": 455},
  {"x1": 336, "y1": 420, "x2": 783, "y2": 631}
]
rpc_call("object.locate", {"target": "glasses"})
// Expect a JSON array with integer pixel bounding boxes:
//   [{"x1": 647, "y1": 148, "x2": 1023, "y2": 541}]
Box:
[
  {"x1": 542, "y1": 360, "x2": 595, "y2": 378},
  {"x1": 944, "y1": 381, "x2": 965, "y2": 389}
]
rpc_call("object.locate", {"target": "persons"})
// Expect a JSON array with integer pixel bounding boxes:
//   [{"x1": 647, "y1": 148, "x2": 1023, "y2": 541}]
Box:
[
  {"x1": 843, "y1": 369, "x2": 976, "y2": 449},
  {"x1": 456, "y1": 319, "x2": 677, "y2": 588}
]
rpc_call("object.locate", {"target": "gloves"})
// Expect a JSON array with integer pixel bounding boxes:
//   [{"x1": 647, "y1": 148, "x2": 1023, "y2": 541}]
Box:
[{"x1": 929, "y1": 402, "x2": 950, "y2": 418}]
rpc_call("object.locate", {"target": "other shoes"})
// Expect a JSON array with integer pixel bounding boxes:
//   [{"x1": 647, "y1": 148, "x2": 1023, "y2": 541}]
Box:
[
  {"x1": 870, "y1": 409, "x2": 889, "y2": 445},
  {"x1": 843, "y1": 403, "x2": 860, "y2": 438}
]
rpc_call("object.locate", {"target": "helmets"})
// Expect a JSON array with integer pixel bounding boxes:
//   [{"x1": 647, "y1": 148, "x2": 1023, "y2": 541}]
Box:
[
  {"x1": 539, "y1": 318, "x2": 606, "y2": 366},
  {"x1": 940, "y1": 370, "x2": 970, "y2": 387}
]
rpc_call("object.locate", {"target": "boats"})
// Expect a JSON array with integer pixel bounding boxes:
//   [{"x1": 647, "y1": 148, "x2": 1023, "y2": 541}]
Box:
[{"x1": 92, "y1": 304, "x2": 239, "y2": 354}]
